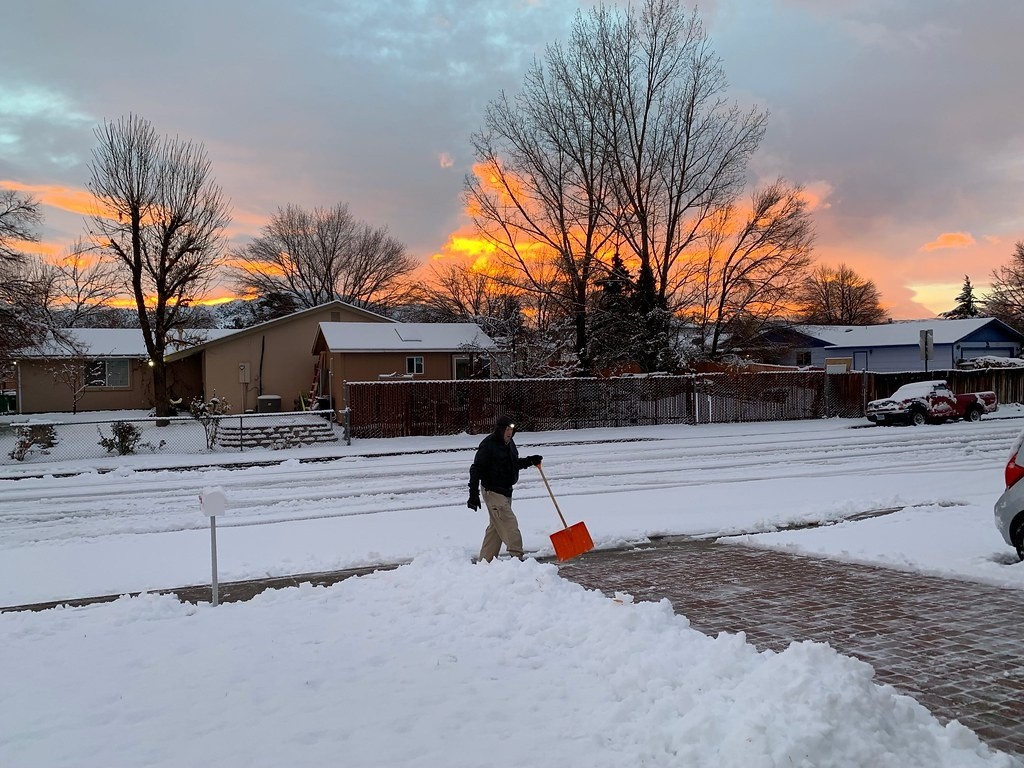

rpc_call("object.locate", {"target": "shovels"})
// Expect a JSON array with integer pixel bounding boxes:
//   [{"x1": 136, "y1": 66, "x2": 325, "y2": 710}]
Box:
[{"x1": 535, "y1": 465, "x2": 595, "y2": 562}]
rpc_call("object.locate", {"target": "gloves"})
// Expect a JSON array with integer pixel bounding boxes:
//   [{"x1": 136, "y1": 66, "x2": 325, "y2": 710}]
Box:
[
  {"x1": 527, "y1": 455, "x2": 543, "y2": 467},
  {"x1": 467, "y1": 489, "x2": 481, "y2": 512}
]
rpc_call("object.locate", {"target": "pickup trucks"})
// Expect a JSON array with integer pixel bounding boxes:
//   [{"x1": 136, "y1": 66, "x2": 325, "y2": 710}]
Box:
[{"x1": 866, "y1": 380, "x2": 999, "y2": 427}]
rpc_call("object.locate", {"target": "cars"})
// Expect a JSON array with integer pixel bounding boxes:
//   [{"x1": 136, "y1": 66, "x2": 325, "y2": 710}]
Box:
[{"x1": 994, "y1": 426, "x2": 1024, "y2": 561}]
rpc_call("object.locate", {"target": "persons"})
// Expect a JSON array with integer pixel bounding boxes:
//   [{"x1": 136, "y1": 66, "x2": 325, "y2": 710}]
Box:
[{"x1": 467, "y1": 416, "x2": 544, "y2": 563}]
